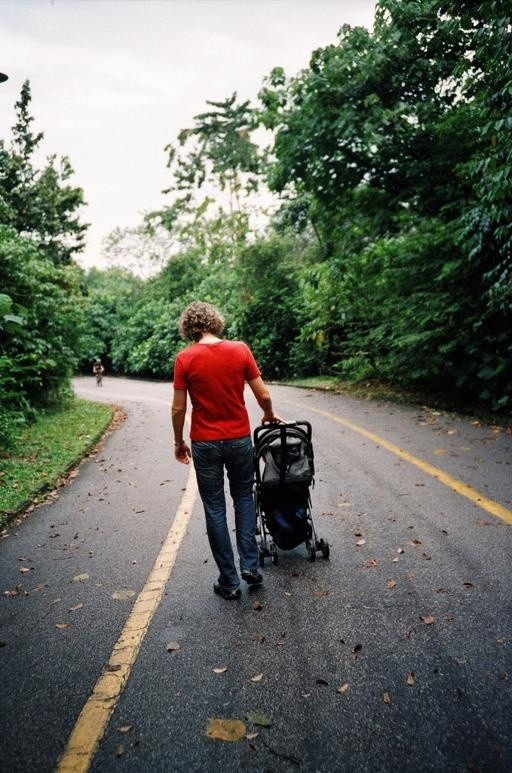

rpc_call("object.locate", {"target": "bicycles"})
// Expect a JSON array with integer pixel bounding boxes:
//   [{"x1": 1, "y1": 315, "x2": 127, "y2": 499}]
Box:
[{"x1": 96, "y1": 370, "x2": 103, "y2": 387}]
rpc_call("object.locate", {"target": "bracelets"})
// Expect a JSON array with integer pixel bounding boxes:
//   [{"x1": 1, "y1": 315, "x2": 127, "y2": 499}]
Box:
[{"x1": 173, "y1": 440, "x2": 184, "y2": 446}]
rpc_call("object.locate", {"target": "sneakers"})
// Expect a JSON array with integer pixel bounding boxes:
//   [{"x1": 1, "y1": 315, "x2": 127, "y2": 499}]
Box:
[
  {"x1": 213, "y1": 581, "x2": 241, "y2": 600},
  {"x1": 242, "y1": 569, "x2": 263, "y2": 584}
]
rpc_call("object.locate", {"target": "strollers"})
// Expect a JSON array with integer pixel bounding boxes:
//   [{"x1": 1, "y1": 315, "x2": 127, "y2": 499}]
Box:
[{"x1": 253, "y1": 420, "x2": 329, "y2": 568}]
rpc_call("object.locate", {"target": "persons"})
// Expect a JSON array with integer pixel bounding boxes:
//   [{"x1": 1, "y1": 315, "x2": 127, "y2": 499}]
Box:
[
  {"x1": 172, "y1": 301, "x2": 286, "y2": 600},
  {"x1": 93, "y1": 359, "x2": 104, "y2": 382}
]
